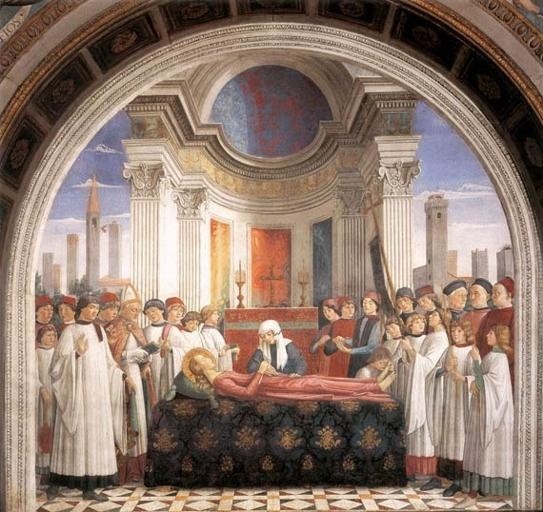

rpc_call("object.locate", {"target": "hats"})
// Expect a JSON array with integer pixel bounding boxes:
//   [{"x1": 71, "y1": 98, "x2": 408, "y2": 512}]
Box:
[
  {"x1": 322, "y1": 296, "x2": 354, "y2": 311},
  {"x1": 396, "y1": 287, "x2": 414, "y2": 299},
  {"x1": 100, "y1": 292, "x2": 118, "y2": 305},
  {"x1": 57, "y1": 295, "x2": 76, "y2": 307},
  {"x1": 119, "y1": 284, "x2": 140, "y2": 308},
  {"x1": 415, "y1": 284, "x2": 434, "y2": 300},
  {"x1": 443, "y1": 279, "x2": 466, "y2": 295},
  {"x1": 166, "y1": 297, "x2": 187, "y2": 313},
  {"x1": 472, "y1": 278, "x2": 493, "y2": 294},
  {"x1": 364, "y1": 291, "x2": 382, "y2": 305},
  {"x1": 499, "y1": 277, "x2": 514, "y2": 298},
  {"x1": 35, "y1": 294, "x2": 53, "y2": 309}
]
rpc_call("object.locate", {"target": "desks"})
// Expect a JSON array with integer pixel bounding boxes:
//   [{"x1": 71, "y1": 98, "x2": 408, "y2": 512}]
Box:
[{"x1": 223, "y1": 305, "x2": 321, "y2": 375}]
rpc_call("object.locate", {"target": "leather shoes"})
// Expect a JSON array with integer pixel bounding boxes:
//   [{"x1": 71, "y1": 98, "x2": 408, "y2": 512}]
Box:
[
  {"x1": 83, "y1": 489, "x2": 109, "y2": 502},
  {"x1": 406, "y1": 473, "x2": 416, "y2": 480},
  {"x1": 421, "y1": 478, "x2": 441, "y2": 490},
  {"x1": 41, "y1": 474, "x2": 59, "y2": 500},
  {"x1": 469, "y1": 489, "x2": 477, "y2": 497},
  {"x1": 443, "y1": 485, "x2": 461, "y2": 496}
]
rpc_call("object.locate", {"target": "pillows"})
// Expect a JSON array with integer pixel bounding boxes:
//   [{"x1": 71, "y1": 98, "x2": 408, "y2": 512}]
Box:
[{"x1": 167, "y1": 371, "x2": 220, "y2": 402}]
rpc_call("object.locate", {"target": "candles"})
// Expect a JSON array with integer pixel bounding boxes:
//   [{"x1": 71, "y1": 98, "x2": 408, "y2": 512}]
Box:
[{"x1": 237, "y1": 258, "x2": 241, "y2": 272}]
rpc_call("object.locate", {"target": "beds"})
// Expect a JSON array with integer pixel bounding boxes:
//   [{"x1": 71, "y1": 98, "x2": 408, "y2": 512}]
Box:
[{"x1": 145, "y1": 390, "x2": 406, "y2": 493}]
